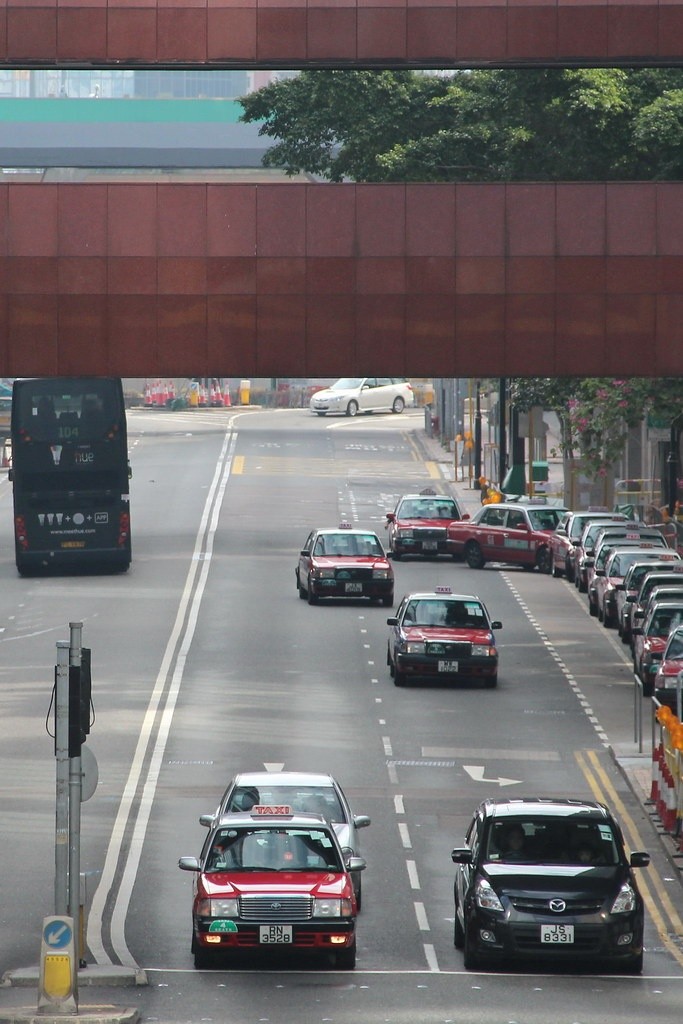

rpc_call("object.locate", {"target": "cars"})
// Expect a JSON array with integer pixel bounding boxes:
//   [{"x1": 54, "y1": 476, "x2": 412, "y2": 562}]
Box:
[
  {"x1": 451, "y1": 797, "x2": 650, "y2": 974},
  {"x1": 296, "y1": 488, "x2": 683, "y2": 725},
  {"x1": 310, "y1": 378, "x2": 414, "y2": 416},
  {"x1": 179, "y1": 772, "x2": 371, "y2": 970}
]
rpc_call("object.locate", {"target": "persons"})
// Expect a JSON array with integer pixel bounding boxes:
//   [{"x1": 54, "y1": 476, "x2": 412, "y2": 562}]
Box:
[{"x1": 498, "y1": 824, "x2": 528, "y2": 861}]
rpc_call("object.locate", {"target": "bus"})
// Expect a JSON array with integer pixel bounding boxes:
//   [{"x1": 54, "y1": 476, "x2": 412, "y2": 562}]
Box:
[{"x1": 8, "y1": 376, "x2": 132, "y2": 577}]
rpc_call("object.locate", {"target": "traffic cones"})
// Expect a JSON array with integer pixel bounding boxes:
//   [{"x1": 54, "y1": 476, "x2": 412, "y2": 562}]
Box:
[{"x1": 144, "y1": 378, "x2": 231, "y2": 407}]
